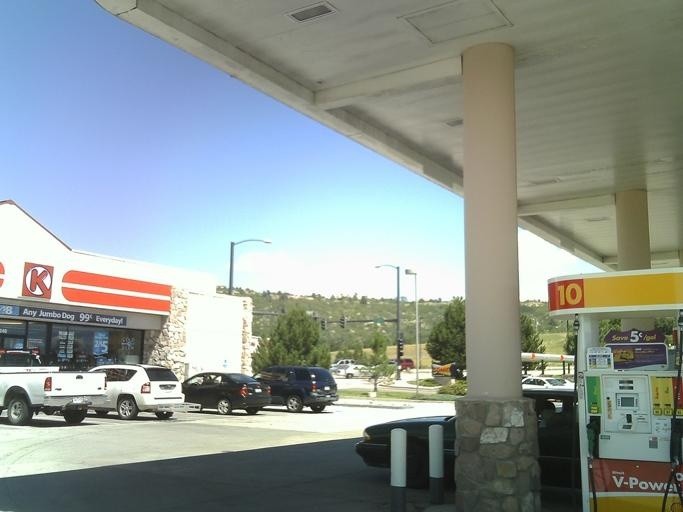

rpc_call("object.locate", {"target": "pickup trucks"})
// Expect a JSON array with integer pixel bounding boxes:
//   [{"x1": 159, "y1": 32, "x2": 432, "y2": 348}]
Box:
[{"x1": 0, "y1": 348, "x2": 110, "y2": 424}]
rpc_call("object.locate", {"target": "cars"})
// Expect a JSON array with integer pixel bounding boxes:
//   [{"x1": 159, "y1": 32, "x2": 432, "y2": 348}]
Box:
[
  {"x1": 521, "y1": 376, "x2": 574, "y2": 402},
  {"x1": 336, "y1": 364, "x2": 375, "y2": 380},
  {"x1": 354, "y1": 390, "x2": 683, "y2": 491},
  {"x1": 330, "y1": 359, "x2": 355, "y2": 368},
  {"x1": 181, "y1": 373, "x2": 272, "y2": 418}
]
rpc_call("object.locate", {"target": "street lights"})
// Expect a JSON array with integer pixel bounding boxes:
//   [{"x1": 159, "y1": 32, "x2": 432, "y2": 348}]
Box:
[
  {"x1": 227, "y1": 238, "x2": 272, "y2": 294},
  {"x1": 376, "y1": 263, "x2": 400, "y2": 380},
  {"x1": 404, "y1": 269, "x2": 419, "y2": 396}
]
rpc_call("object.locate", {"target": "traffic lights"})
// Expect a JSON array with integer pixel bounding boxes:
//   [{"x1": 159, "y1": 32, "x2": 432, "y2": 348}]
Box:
[
  {"x1": 321, "y1": 320, "x2": 324, "y2": 329},
  {"x1": 399, "y1": 339, "x2": 403, "y2": 350},
  {"x1": 340, "y1": 317, "x2": 343, "y2": 327}
]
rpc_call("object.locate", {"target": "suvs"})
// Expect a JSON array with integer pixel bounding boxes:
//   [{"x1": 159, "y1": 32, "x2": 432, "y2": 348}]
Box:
[
  {"x1": 81, "y1": 363, "x2": 186, "y2": 423},
  {"x1": 251, "y1": 363, "x2": 338, "y2": 416},
  {"x1": 385, "y1": 358, "x2": 413, "y2": 371}
]
